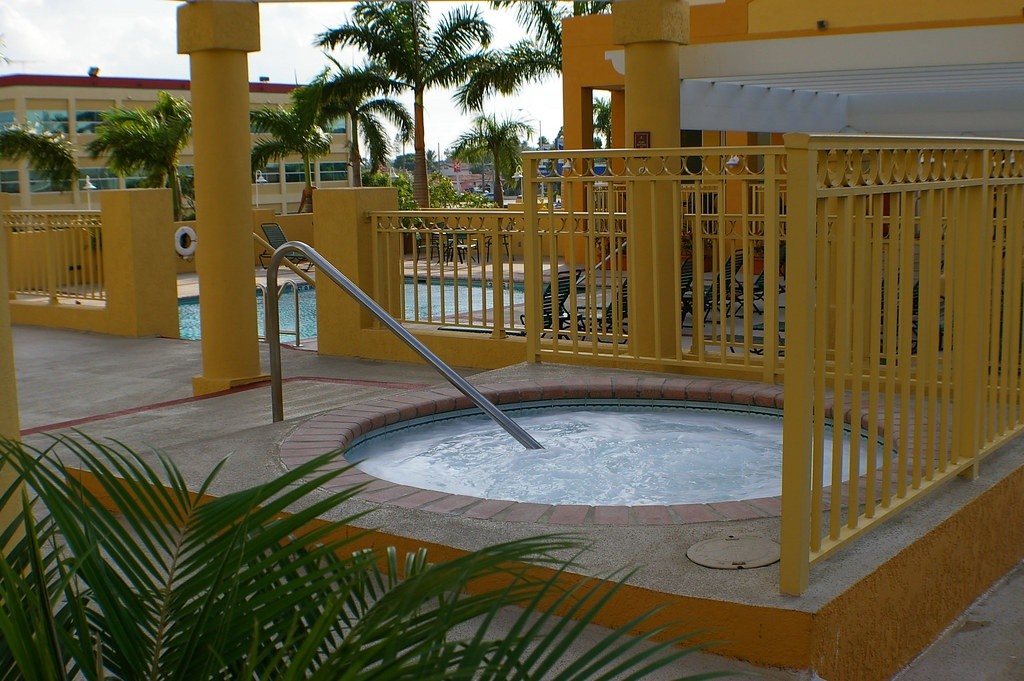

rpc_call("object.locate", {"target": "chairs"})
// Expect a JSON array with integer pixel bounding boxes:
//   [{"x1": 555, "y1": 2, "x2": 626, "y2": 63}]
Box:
[
  {"x1": 415, "y1": 222, "x2": 515, "y2": 267},
  {"x1": 260, "y1": 222, "x2": 314, "y2": 272},
  {"x1": 523, "y1": 246, "x2": 786, "y2": 344}
]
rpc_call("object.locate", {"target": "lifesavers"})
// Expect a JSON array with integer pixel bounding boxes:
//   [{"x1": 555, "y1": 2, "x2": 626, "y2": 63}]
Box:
[{"x1": 175, "y1": 227, "x2": 197, "y2": 256}]
